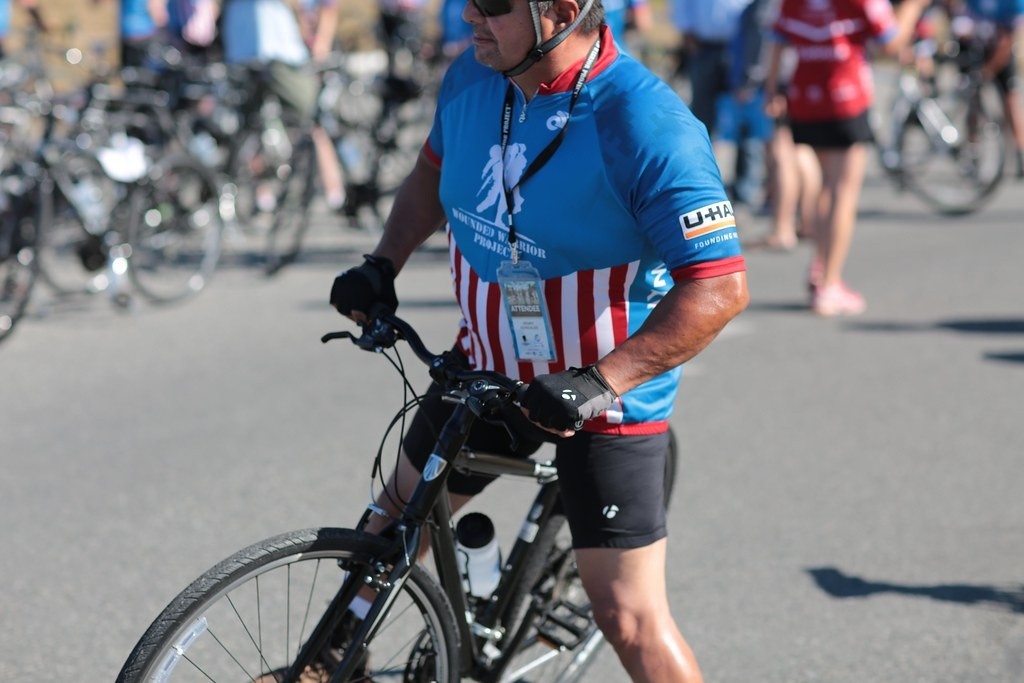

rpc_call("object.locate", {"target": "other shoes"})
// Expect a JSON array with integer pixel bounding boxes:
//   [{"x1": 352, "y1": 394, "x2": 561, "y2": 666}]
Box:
[
  {"x1": 808, "y1": 255, "x2": 826, "y2": 289},
  {"x1": 250, "y1": 641, "x2": 371, "y2": 683},
  {"x1": 813, "y1": 279, "x2": 865, "y2": 315}
]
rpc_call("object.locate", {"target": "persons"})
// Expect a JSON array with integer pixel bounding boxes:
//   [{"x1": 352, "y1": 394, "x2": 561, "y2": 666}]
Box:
[
  {"x1": 116, "y1": 0, "x2": 477, "y2": 214},
  {"x1": 603, "y1": 0, "x2": 931, "y2": 314},
  {"x1": 895, "y1": 0, "x2": 1024, "y2": 180},
  {"x1": 254, "y1": 0, "x2": 749, "y2": 683}
]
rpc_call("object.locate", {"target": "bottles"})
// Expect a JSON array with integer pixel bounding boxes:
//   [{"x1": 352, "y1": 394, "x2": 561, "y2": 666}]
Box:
[
  {"x1": 69, "y1": 172, "x2": 104, "y2": 228},
  {"x1": 456, "y1": 513, "x2": 502, "y2": 600}
]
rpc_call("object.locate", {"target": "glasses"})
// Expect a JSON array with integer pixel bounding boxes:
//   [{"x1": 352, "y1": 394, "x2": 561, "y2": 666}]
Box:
[{"x1": 472, "y1": 0, "x2": 512, "y2": 17}]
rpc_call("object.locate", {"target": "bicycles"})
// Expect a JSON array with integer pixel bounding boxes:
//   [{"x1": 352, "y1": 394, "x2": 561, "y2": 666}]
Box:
[
  {"x1": 115, "y1": 304, "x2": 679, "y2": 682},
  {"x1": 0, "y1": 14, "x2": 1011, "y2": 345}
]
